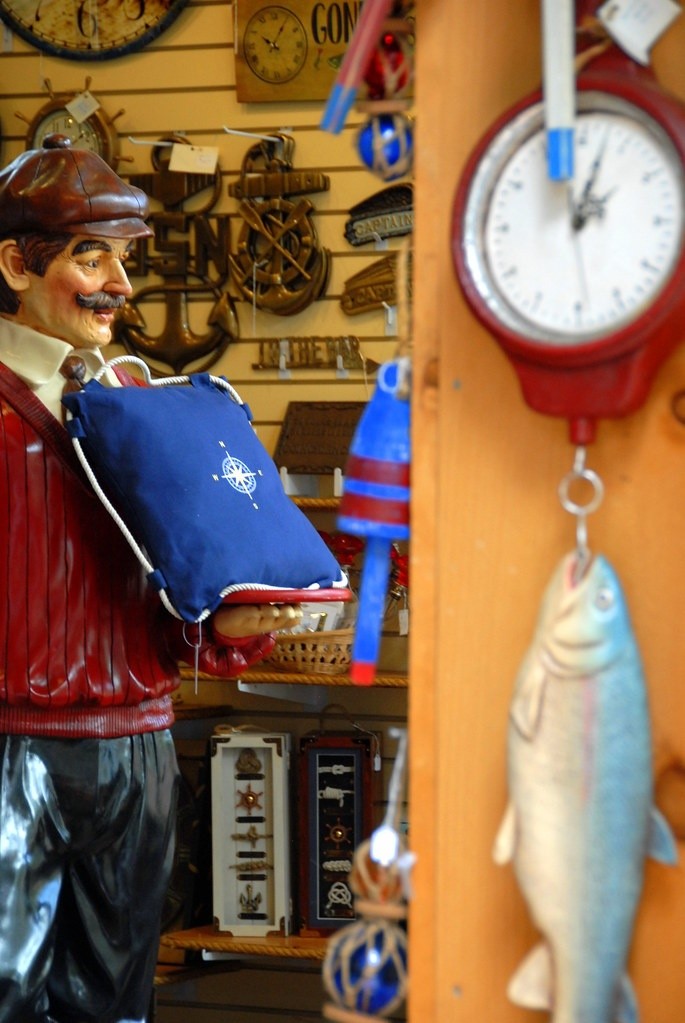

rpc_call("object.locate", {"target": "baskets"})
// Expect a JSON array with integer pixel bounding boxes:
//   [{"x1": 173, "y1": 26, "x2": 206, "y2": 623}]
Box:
[{"x1": 267, "y1": 627, "x2": 355, "y2": 676}]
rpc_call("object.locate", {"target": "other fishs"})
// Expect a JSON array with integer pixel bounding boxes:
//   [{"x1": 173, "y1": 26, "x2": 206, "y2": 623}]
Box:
[{"x1": 491, "y1": 545, "x2": 675, "y2": 1023}]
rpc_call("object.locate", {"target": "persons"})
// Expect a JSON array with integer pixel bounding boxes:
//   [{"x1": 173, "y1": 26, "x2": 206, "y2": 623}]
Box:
[{"x1": 0, "y1": 133, "x2": 303, "y2": 1023}]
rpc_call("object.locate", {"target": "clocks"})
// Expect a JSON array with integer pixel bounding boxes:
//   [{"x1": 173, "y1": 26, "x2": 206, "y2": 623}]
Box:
[{"x1": 0, "y1": 0, "x2": 306, "y2": 172}]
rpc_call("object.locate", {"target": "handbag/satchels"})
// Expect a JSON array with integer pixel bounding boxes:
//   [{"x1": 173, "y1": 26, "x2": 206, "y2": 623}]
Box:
[{"x1": 67, "y1": 358, "x2": 350, "y2": 627}]
